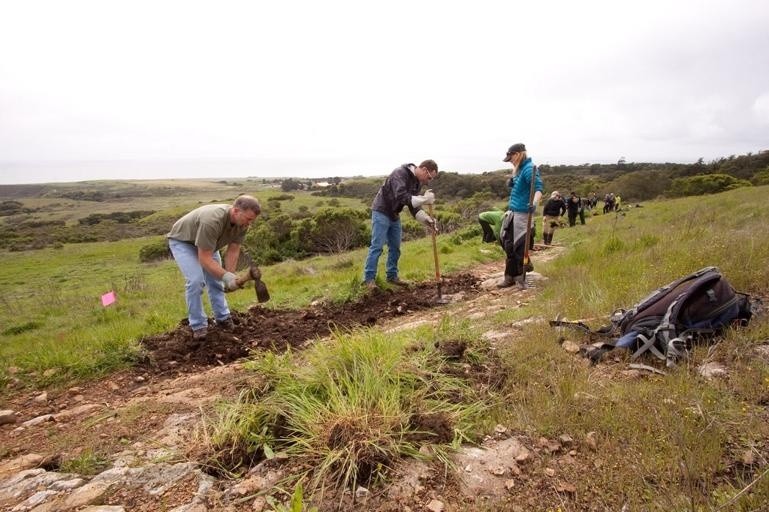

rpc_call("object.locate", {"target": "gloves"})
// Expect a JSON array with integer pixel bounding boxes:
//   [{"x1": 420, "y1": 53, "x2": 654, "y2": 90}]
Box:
[
  {"x1": 412, "y1": 189, "x2": 435, "y2": 210},
  {"x1": 526, "y1": 201, "x2": 537, "y2": 214},
  {"x1": 222, "y1": 272, "x2": 238, "y2": 290},
  {"x1": 416, "y1": 208, "x2": 438, "y2": 232}
]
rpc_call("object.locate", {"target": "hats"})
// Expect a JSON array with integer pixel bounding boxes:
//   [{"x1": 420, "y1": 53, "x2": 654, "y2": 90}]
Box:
[
  {"x1": 503, "y1": 144, "x2": 526, "y2": 163},
  {"x1": 551, "y1": 191, "x2": 559, "y2": 199}
]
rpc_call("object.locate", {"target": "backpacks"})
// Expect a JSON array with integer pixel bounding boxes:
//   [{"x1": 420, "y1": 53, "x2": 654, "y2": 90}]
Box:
[{"x1": 611, "y1": 266, "x2": 753, "y2": 359}]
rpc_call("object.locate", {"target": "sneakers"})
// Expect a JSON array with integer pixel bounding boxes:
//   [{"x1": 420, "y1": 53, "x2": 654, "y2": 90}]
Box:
[
  {"x1": 365, "y1": 279, "x2": 377, "y2": 288},
  {"x1": 386, "y1": 274, "x2": 407, "y2": 286},
  {"x1": 216, "y1": 318, "x2": 236, "y2": 331},
  {"x1": 193, "y1": 329, "x2": 207, "y2": 340}
]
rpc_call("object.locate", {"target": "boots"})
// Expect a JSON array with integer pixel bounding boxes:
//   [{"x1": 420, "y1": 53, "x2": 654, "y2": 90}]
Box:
[
  {"x1": 498, "y1": 274, "x2": 527, "y2": 289},
  {"x1": 544, "y1": 233, "x2": 554, "y2": 245}
]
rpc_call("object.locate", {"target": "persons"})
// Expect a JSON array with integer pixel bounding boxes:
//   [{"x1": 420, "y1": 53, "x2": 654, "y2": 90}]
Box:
[
  {"x1": 566, "y1": 191, "x2": 582, "y2": 228},
  {"x1": 614, "y1": 192, "x2": 622, "y2": 211},
  {"x1": 496, "y1": 143, "x2": 544, "y2": 289},
  {"x1": 477, "y1": 210, "x2": 510, "y2": 242},
  {"x1": 603, "y1": 194, "x2": 610, "y2": 214},
  {"x1": 609, "y1": 192, "x2": 615, "y2": 212},
  {"x1": 360, "y1": 159, "x2": 440, "y2": 292},
  {"x1": 165, "y1": 194, "x2": 261, "y2": 341},
  {"x1": 542, "y1": 190, "x2": 567, "y2": 246},
  {"x1": 591, "y1": 193, "x2": 599, "y2": 208}
]
